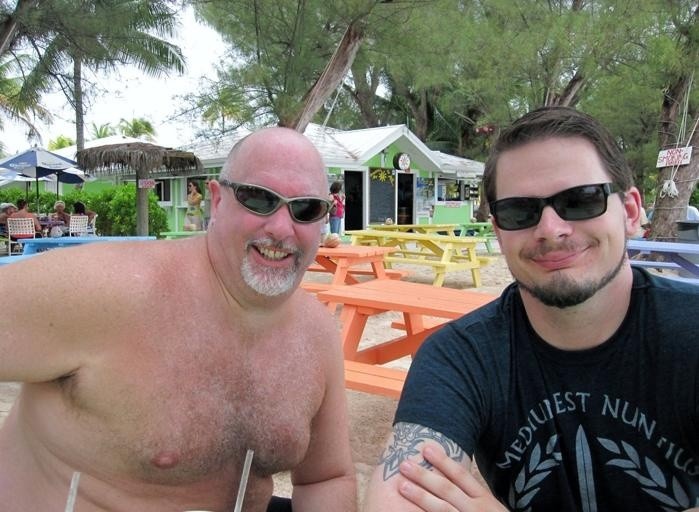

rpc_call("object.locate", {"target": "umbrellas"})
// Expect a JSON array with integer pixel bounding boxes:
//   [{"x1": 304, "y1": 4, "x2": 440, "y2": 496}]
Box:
[
  {"x1": 0, "y1": 144, "x2": 97, "y2": 215},
  {"x1": 74, "y1": 142, "x2": 204, "y2": 236}
]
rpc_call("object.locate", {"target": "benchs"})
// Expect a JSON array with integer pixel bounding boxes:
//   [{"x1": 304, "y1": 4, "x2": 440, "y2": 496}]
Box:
[
  {"x1": 307, "y1": 224, "x2": 498, "y2": 402},
  {"x1": 620, "y1": 232, "x2": 699, "y2": 284}
]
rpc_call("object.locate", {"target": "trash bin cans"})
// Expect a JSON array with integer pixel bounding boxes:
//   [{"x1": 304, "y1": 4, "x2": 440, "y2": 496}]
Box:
[{"x1": 675, "y1": 220, "x2": 699, "y2": 279}]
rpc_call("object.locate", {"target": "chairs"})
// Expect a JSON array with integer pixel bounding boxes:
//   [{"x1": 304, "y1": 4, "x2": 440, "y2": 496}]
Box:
[{"x1": 0, "y1": 211, "x2": 98, "y2": 256}]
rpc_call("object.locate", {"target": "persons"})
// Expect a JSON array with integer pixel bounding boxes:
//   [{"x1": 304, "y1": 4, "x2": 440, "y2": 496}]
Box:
[
  {"x1": 0, "y1": 199, "x2": 89, "y2": 253},
  {"x1": 363, "y1": 107, "x2": 699, "y2": 512},
  {"x1": 0, "y1": 126, "x2": 357, "y2": 512},
  {"x1": 631, "y1": 208, "x2": 651, "y2": 237},
  {"x1": 184, "y1": 181, "x2": 205, "y2": 231},
  {"x1": 326, "y1": 182, "x2": 346, "y2": 233}
]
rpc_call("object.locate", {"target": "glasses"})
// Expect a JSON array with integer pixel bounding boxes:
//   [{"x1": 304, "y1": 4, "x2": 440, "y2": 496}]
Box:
[
  {"x1": 488, "y1": 181, "x2": 618, "y2": 231},
  {"x1": 219, "y1": 179, "x2": 336, "y2": 225}
]
